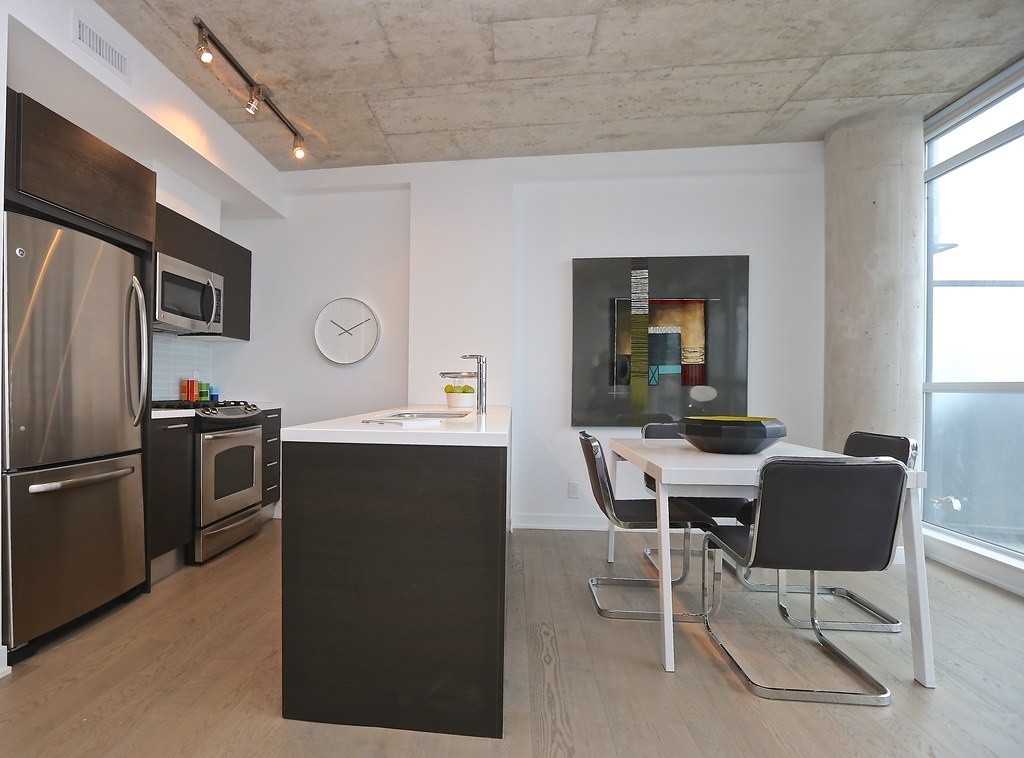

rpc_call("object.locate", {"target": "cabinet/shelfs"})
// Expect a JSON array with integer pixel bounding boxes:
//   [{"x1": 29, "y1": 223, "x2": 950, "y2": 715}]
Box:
[
  {"x1": 4, "y1": 87, "x2": 252, "y2": 341},
  {"x1": 141, "y1": 408, "x2": 281, "y2": 593}
]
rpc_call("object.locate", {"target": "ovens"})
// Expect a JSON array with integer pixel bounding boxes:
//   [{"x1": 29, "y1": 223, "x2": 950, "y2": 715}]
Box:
[{"x1": 195, "y1": 424, "x2": 262, "y2": 563}]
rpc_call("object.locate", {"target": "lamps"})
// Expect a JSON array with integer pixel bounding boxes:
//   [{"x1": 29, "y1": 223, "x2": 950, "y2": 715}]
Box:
[
  {"x1": 196, "y1": 28, "x2": 213, "y2": 63},
  {"x1": 293, "y1": 136, "x2": 304, "y2": 159},
  {"x1": 245, "y1": 84, "x2": 274, "y2": 115}
]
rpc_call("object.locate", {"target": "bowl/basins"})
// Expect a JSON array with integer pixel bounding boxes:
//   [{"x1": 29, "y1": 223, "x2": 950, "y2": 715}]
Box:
[{"x1": 446, "y1": 393, "x2": 476, "y2": 408}]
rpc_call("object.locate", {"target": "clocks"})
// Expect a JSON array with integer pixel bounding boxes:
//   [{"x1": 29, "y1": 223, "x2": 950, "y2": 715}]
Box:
[{"x1": 313, "y1": 297, "x2": 380, "y2": 365}]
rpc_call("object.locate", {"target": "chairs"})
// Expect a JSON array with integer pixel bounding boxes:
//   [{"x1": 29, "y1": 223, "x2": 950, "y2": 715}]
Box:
[
  {"x1": 579, "y1": 431, "x2": 722, "y2": 622},
  {"x1": 702, "y1": 456, "x2": 908, "y2": 706},
  {"x1": 736, "y1": 430, "x2": 919, "y2": 633},
  {"x1": 642, "y1": 422, "x2": 752, "y2": 581}
]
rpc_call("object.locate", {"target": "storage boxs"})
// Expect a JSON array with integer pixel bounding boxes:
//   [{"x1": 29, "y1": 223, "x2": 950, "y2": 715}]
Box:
[{"x1": 446, "y1": 392, "x2": 477, "y2": 408}]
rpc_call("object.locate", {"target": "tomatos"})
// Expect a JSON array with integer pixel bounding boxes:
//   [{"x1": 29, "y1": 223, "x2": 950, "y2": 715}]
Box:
[{"x1": 444, "y1": 385, "x2": 475, "y2": 393}]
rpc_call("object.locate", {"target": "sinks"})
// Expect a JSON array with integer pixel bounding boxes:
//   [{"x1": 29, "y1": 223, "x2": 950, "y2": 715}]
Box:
[{"x1": 376, "y1": 411, "x2": 471, "y2": 419}]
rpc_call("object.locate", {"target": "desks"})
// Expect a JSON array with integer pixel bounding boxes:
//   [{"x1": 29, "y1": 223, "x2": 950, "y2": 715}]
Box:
[{"x1": 608, "y1": 437, "x2": 937, "y2": 689}]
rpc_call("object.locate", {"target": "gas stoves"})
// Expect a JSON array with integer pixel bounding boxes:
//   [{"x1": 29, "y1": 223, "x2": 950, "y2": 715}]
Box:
[{"x1": 151, "y1": 401, "x2": 261, "y2": 432}]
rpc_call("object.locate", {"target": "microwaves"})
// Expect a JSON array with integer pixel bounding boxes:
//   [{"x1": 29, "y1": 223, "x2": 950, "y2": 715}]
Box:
[{"x1": 154, "y1": 252, "x2": 223, "y2": 336}]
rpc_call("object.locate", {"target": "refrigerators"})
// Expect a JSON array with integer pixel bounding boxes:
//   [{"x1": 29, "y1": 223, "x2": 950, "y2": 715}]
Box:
[{"x1": 3, "y1": 209, "x2": 154, "y2": 666}]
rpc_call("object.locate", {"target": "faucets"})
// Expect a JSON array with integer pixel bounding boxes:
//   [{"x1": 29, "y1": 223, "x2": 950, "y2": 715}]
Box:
[{"x1": 438, "y1": 355, "x2": 488, "y2": 416}]
